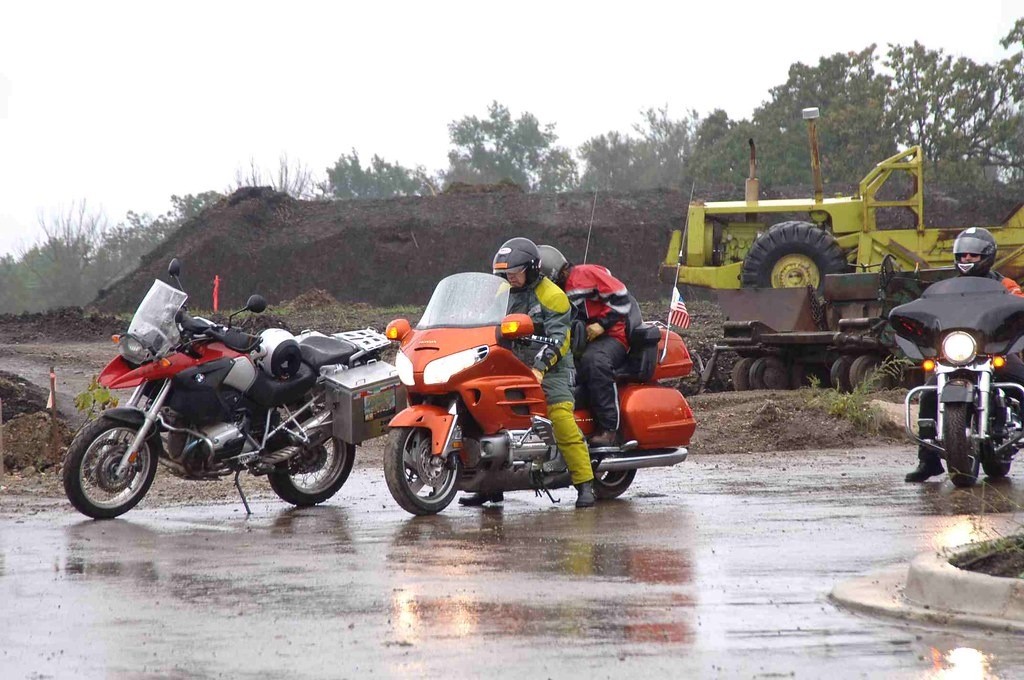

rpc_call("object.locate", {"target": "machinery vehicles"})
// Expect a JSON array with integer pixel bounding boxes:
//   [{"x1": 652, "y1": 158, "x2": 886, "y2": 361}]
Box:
[{"x1": 659, "y1": 105, "x2": 1023, "y2": 393}]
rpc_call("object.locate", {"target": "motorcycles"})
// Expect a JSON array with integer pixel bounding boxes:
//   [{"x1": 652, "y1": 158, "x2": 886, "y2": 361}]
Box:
[
  {"x1": 60, "y1": 255, "x2": 406, "y2": 519},
  {"x1": 889, "y1": 275, "x2": 1024, "y2": 488},
  {"x1": 384, "y1": 273, "x2": 697, "y2": 516}
]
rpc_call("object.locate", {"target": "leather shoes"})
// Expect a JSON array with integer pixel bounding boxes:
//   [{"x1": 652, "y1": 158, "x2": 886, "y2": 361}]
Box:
[
  {"x1": 573, "y1": 481, "x2": 598, "y2": 507},
  {"x1": 457, "y1": 490, "x2": 504, "y2": 506},
  {"x1": 904, "y1": 459, "x2": 945, "y2": 483}
]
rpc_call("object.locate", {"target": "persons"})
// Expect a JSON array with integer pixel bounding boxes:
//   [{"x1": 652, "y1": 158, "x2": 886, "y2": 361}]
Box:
[
  {"x1": 904, "y1": 226, "x2": 1024, "y2": 485},
  {"x1": 532, "y1": 242, "x2": 632, "y2": 446},
  {"x1": 457, "y1": 237, "x2": 597, "y2": 507}
]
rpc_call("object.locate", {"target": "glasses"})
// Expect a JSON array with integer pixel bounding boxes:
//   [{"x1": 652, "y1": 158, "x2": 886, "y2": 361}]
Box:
[{"x1": 958, "y1": 253, "x2": 979, "y2": 258}]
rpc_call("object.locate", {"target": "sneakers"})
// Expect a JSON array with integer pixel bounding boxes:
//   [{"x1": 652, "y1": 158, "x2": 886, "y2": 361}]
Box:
[{"x1": 588, "y1": 428, "x2": 625, "y2": 447}]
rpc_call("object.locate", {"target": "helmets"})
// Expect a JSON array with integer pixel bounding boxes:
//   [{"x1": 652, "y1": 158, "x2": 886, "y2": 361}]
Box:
[
  {"x1": 535, "y1": 244, "x2": 568, "y2": 284},
  {"x1": 251, "y1": 327, "x2": 302, "y2": 380},
  {"x1": 492, "y1": 237, "x2": 541, "y2": 292},
  {"x1": 952, "y1": 226, "x2": 998, "y2": 276}
]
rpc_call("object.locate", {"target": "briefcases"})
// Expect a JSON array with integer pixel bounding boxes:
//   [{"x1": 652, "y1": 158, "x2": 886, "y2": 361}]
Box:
[{"x1": 324, "y1": 360, "x2": 409, "y2": 445}]
